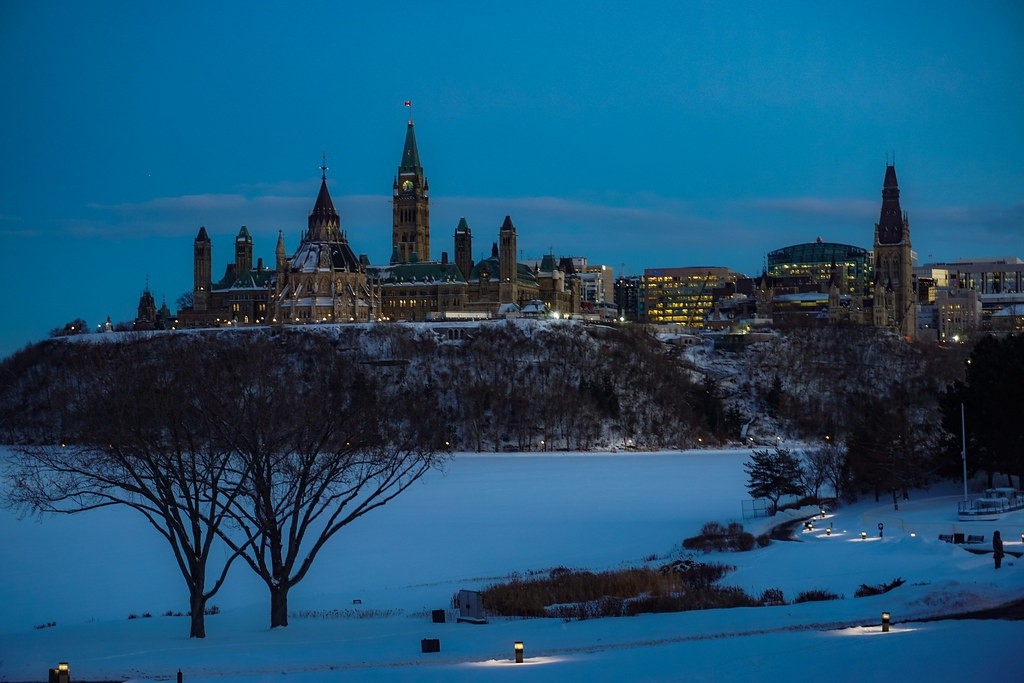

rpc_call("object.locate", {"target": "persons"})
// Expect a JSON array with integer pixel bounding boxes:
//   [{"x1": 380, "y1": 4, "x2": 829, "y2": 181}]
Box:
[{"x1": 992, "y1": 530, "x2": 1005, "y2": 569}]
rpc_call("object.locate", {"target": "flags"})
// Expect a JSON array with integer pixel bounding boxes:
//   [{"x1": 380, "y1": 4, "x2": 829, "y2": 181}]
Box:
[{"x1": 405, "y1": 101, "x2": 411, "y2": 106}]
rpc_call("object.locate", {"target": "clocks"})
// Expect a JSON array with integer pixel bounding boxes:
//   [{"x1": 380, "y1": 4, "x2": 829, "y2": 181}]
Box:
[{"x1": 402, "y1": 179, "x2": 414, "y2": 194}]
